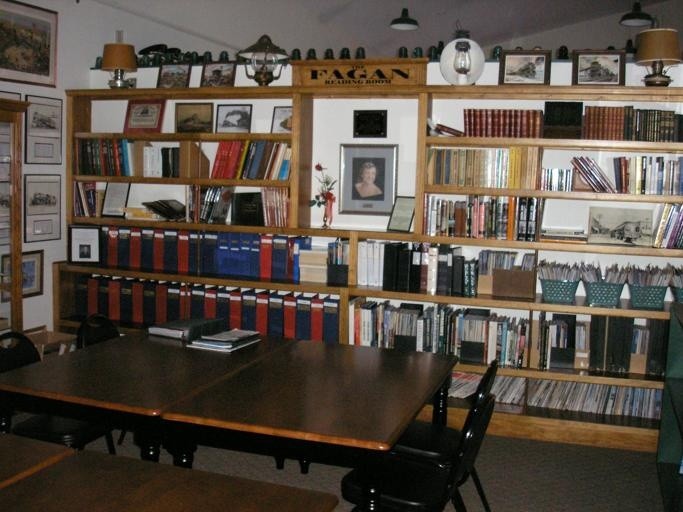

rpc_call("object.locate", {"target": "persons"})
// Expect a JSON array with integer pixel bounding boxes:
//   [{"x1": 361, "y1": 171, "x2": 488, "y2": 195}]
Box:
[
  {"x1": 354, "y1": 161, "x2": 385, "y2": 199},
  {"x1": 205, "y1": 68, "x2": 231, "y2": 88}
]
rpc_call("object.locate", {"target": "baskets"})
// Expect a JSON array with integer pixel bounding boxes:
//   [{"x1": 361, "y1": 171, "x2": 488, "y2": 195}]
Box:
[
  {"x1": 540, "y1": 279, "x2": 579, "y2": 304},
  {"x1": 670, "y1": 285, "x2": 683, "y2": 302},
  {"x1": 584, "y1": 281, "x2": 624, "y2": 307},
  {"x1": 628, "y1": 282, "x2": 668, "y2": 309}
]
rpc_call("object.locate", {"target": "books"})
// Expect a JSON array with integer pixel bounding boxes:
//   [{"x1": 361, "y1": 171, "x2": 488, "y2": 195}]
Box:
[
  {"x1": 348, "y1": 297, "x2": 529, "y2": 368},
  {"x1": 529, "y1": 311, "x2": 671, "y2": 375},
  {"x1": 326, "y1": 235, "x2": 348, "y2": 266},
  {"x1": 71, "y1": 134, "x2": 294, "y2": 228},
  {"x1": 538, "y1": 259, "x2": 683, "y2": 305},
  {"x1": 417, "y1": 100, "x2": 682, "y2": 248},
  {"x1": 185, "y1": 325, "x2": 264, "y2": 354},
  {"x1": 447, "y1": 371, "x2": 526, "y2": 413},
  {"x1": 358, "y1": 241, "x2": 535, "y2": 298},
  {"x1": 528, "y1": 379, "x2": 661, "y2": 420}
]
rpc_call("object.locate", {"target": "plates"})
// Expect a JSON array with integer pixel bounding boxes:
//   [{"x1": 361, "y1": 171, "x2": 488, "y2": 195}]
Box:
[{"x1": 440, "y1": 39, "x2": 485, "y2": 86}]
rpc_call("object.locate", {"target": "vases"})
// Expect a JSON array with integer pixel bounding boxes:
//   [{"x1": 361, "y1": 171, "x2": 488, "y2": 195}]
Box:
[{"x1": 322, "y1": 199, "x2": 333, "y2": 227}]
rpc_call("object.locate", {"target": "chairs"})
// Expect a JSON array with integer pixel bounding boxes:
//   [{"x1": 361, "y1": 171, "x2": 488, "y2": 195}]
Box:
[
  {"x1": 340, "y1": 358, "x2": 498, "y2": 511},
  {"x1": 75, "y1": 314, "x2": 133, "y2": 444}
]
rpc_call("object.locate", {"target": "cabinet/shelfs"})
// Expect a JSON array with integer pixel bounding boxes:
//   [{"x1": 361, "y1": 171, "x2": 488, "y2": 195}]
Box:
[
  {"x1": 654, "y1": 301, "x2": 682, "y2": 511},
  {"x1": 65, "y1": 58, "x2": 683, "y2": 453}
]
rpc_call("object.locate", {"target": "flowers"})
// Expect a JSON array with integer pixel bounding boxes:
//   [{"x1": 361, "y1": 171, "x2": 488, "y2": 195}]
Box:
[{"x1": 305, "y1": 159, "x2": 335, "y2": 209}]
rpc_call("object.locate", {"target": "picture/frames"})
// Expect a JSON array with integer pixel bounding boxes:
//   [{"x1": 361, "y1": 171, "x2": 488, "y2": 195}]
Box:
[
  {"x1": 336, "y1": 141, "x2": 414, "y2": 233},
  {"x1": 100, "y1": 183, "x2": 131, "y2": 218},
  {"x1": 122, "y1": 98, "x2": 293, "y2": 135},
  {"x1": 155, "y1": 62, "x2": 237, "y2": 89},
  {"x1": 68, "y1": 224, "x2": 101, "y2": 265},
  {"x1": 0, "y1": 1, "x2": 62, "y2": 301},
  {"x1": 497, "y1": 48, "x2": 627, "y2": 87}
]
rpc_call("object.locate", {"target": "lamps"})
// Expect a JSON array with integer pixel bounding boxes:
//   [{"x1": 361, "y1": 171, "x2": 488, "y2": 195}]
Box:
[
  {"x1": 387, "y1": 7, "x2": 419, "y2": 32},
  {"x1": 618, "y1": 3, "x2": 683, "y2": 88},
  {"x1": 437, "y1": 19, "x2": 485, "y2": 86},
  {"x1": 237, "y1": 34, "x2": 290, "y2": 87},
  {"x1": 101, "y1": 31, "x2": 138, "y2": 89}
]
